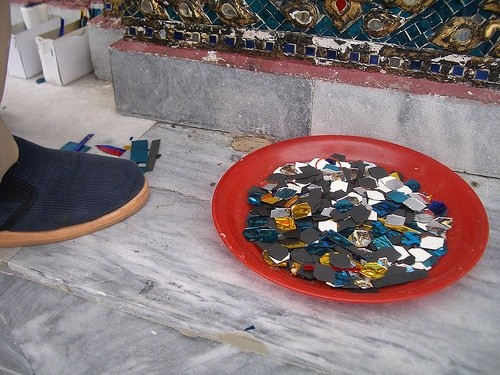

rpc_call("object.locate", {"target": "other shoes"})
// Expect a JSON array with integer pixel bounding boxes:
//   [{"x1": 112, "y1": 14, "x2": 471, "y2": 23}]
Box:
[{"x1": 0, "y1": 135, "x2": 150, "y2": 248}]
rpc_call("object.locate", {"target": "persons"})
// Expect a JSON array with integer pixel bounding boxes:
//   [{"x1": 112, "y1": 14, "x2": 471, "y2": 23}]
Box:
[{"x1": 0, "y1": 1, "x2": 150, "y2": 247}]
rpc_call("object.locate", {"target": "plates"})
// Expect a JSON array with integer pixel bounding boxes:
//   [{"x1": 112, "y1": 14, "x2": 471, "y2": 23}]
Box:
[{"x1": 212, "y1": 134, "x2": 492, "y2": 305}]
